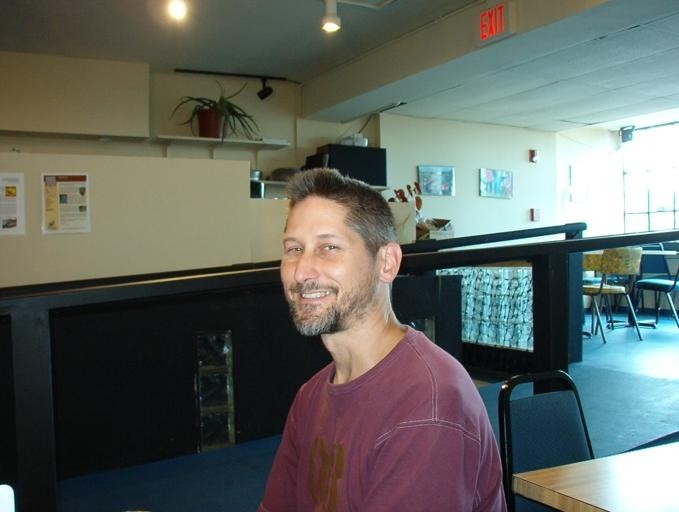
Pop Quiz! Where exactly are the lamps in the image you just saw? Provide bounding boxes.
[320,0,342,33]
[167,0,187,21]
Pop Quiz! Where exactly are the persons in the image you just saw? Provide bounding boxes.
[258,168,508,511]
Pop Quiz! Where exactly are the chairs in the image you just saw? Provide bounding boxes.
[498,370,594,512]
[632,269,679,329]
[582,247,644,344]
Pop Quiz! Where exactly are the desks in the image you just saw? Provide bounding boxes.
[583,250,679,330]
[511,441,678,511]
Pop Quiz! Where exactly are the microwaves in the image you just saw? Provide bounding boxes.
[317,143,387,187]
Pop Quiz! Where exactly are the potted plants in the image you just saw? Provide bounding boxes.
[169,82,260,145]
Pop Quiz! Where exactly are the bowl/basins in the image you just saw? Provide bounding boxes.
[354,137,368,147]
[340,138,354,146]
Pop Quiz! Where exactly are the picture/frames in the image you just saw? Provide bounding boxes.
[417,166,456,196]
[478,167,513,198]
